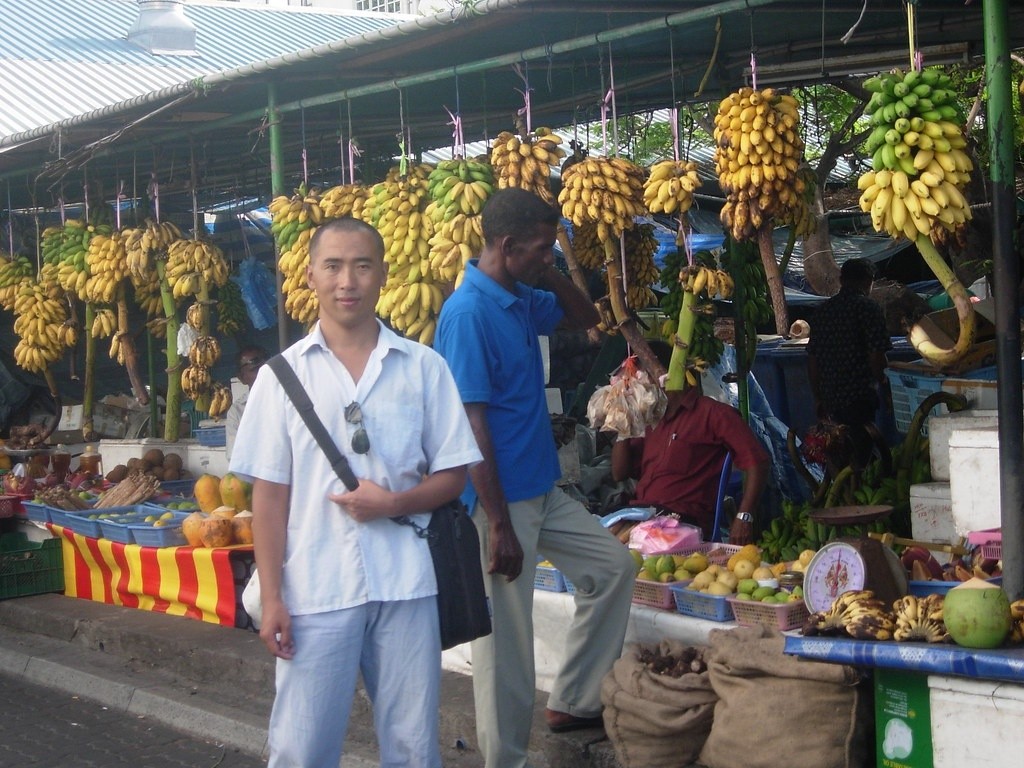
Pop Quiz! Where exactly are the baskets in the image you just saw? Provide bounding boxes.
[529,513,816,630]
[1,467,206,549]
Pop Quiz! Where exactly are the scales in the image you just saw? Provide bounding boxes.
[803,505,908,617]
[3,444,52,479]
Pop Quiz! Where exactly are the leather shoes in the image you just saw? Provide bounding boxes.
[543,706,603,732]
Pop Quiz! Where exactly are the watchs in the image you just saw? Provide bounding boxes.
[736,512,754,523]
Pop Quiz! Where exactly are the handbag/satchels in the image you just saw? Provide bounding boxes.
[425,500,493,649]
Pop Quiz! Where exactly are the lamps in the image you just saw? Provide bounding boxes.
[743,41,979,88]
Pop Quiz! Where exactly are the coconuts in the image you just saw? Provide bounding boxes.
[942,577,1011,649]
[106,449,193,482]
[182,472,254,548]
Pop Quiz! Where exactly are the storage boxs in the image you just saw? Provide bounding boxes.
[536,333,551,385]
[926,674,1024,768]
[57,404,127,439]
[65,504,165,539]
[191,427,226,448]
[545,386,566,415]
[127,517,189,549]
[0,493,18,519]
[909,575,1003,598]
[880,364,997,436]
[533,552,566,593]
[947,424,1001,537]
[871,667,933,768]
[150,498,196,507]
[20,499,50,523]
[635,310,669,343]
[553,427,582,486]
[159,478,194,495]
[928,407,999,481]
[969,526,1003,559]
[667,582,734,623]
[905,307,998,375]
[940,377,998,414]
[96,510,190,544]
[6,492,34,516]
[44,431,84,444]
[561,573,578,594]
[726,591,809,631]
[908,479,958,563]
[633,539,765,610]
[46,500,97,530]
[0,531,66,599]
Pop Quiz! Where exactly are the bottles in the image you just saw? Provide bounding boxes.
[93,474,103,489]
[0,476,5,496]
[79,445,103,484]
[53,443,71,483]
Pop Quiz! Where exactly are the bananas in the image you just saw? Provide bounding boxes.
[799,590,1024,646]
[559,154,776,387]
[266,127,566,348]
[756,435,930,562]
[714,86,819,242]
[0,200,245,416]
[858,69,974,246]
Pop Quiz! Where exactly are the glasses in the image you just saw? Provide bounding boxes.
[239,353,270,371]
[344,399,371,458]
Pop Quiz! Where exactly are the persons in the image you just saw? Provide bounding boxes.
[805,259,893,474]
[225,346,271,462]
[227,218,484,768]
[611,342,770,547]
[434,187,640,768]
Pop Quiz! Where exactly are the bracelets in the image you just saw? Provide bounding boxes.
[554,280,572,295]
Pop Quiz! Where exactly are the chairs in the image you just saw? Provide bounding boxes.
[708,449,735,541]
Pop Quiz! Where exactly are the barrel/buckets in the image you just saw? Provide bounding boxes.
[927,288,977,311]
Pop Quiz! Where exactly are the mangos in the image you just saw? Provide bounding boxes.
[626,545,817,604]
[87,502,199,528]
